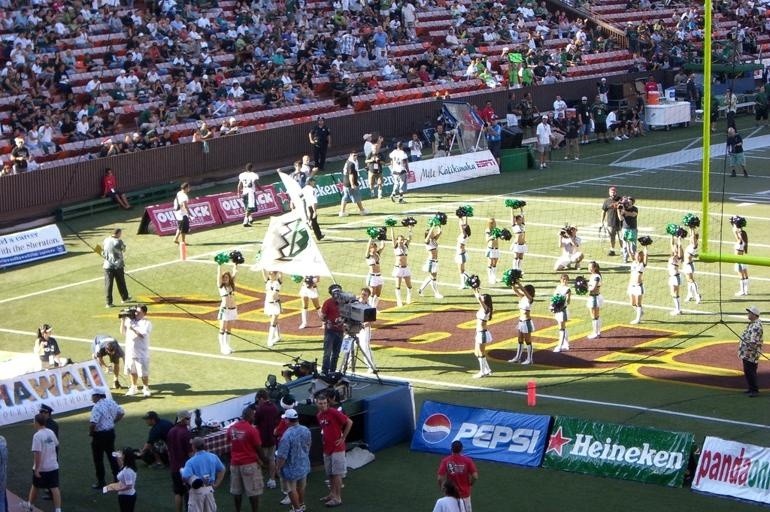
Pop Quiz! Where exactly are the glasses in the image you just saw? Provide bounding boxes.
[353,155,358,156]
[185,416,191,420]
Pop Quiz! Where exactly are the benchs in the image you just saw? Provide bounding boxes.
[718,101,757,121]
[58,180,184,223]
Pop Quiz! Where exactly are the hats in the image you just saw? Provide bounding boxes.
[318,117,325,121]
[542,115,549,119]
[141,411,158,419]
[37,403,54,413]
[40,324,53,333]
[177,410,192,423]
[746,306,761,317]
[363,133,373,141]
[281,409,298,418]
[189,437,205,448]
[89,386,105,394]
[582,96,587,100]
[306,176,316,182]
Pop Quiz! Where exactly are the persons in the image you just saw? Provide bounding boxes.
[237,133,410,241]
[0,1,769,176]
[432,440,478,511]
[217,260,325,355]
[739,307,764,398]
[104,229,132,309]
[601,187,639,261]
[0,389,353,511]
[173,183,194,245]
[367,207,527,314]
[35,324,62,369]
[102,169,134,210]
[473,226,702,379]
[91,303,152,398]
[734,225,750,296]
[321,283,377,378]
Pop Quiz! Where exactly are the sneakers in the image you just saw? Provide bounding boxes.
[142,387,152,396]
[575,158,579,160]
[730,172,736,176]
[539,164,543,171]
[543,163,548,168]
[580,143,585,145]
[339,211,349,217]
[564,157,568,160]
[91,481,107,489]
[121,295,132,303]
[744,173,748,176]
[106,304,115,307]
[399,199,408,204]
[289,505,303,512]
[114,381,121,389]
[391,195,396,203]
[744,389,751,393]
[320,493,335,502]
[279,495,291,504]
[249,219,255,224]
[585,143,590,144]
[371,194,375,198]
[359,208,369,215]
[125,388,138,396]
[318,235,325,240]
[614,136,622,141]
[597,138,601,143]
[378,194,383,199]
[608,250,615,255]
[604,138,611,143]
[621,135,629,140]
[300,504,306,510]
[325,498,342,506]
[18,502,33,512]
[267,479,276,489]
[750,392,760,397]
[243,224,252,227]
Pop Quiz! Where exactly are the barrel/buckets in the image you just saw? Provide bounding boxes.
[665,89,676,102]
[648,91,660,105]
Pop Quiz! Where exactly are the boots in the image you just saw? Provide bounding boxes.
[745,278,749,295]
[268,325,276,347]
[685,282,693,305]
[518,257,525,275]
[396,288,403,307]
[521,343,534,365]
[552,328,566,353]
[512,258,518,269]
[457,274,464,288]
[273,323,281,344]
[492,266,496,284]
[224,332,235,353]
[475,354,492,375]
[373,295,380,314]
[472,355,486,379]
[316,306,326,330]
[369,295,374,308]
[691,282,701,304]
[298,307,309,329]
[562,329,569,351]
[219,332,231,354]
[587,318,598,339]
[507,342,524,363]
[487,266,492,283]
[630,307,642,324]
[418,276,431,295]
[431,278,443,298]
[632,304,644,317]
[735,279,745,296]
[406,287,412,304]
[597,316,601,336]
[670,296,681,316]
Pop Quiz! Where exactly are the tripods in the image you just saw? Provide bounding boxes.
[339,340,387,385]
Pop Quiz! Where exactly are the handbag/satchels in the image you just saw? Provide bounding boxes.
[174,197,180,211]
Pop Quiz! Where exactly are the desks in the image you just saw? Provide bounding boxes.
[644,100,692,132]
[184,424,232,466]
